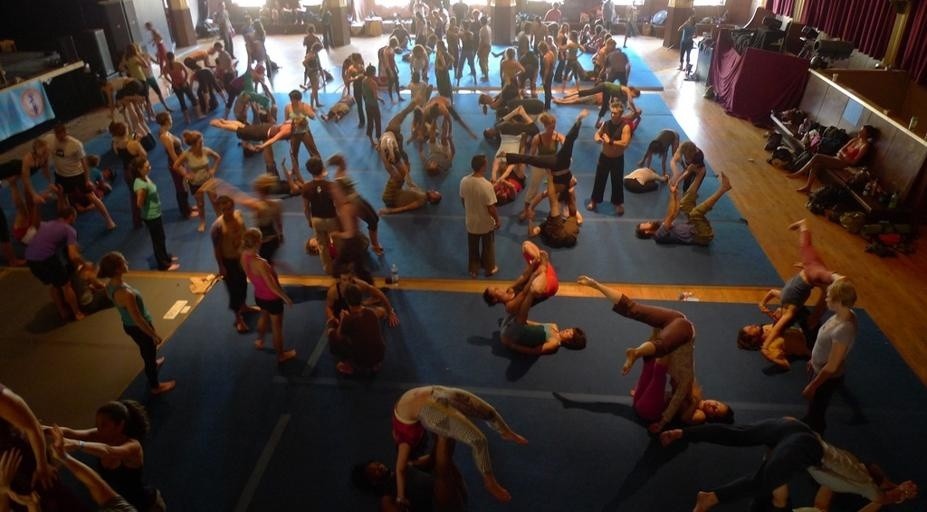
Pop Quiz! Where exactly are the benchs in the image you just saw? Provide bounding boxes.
[350,16,413,35]
[516,19,744,36]
[231,20,320,34]
[769,108,898,215]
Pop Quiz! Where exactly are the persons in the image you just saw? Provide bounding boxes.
[797,282,864,431]
[349,385,530,512]
[2,1,925,373]
[661,416,923,512]
[1,384,166,512]
[577,276,735,445]
[97,250,176,395]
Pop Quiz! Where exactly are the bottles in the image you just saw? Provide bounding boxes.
[848,167,901,210]
